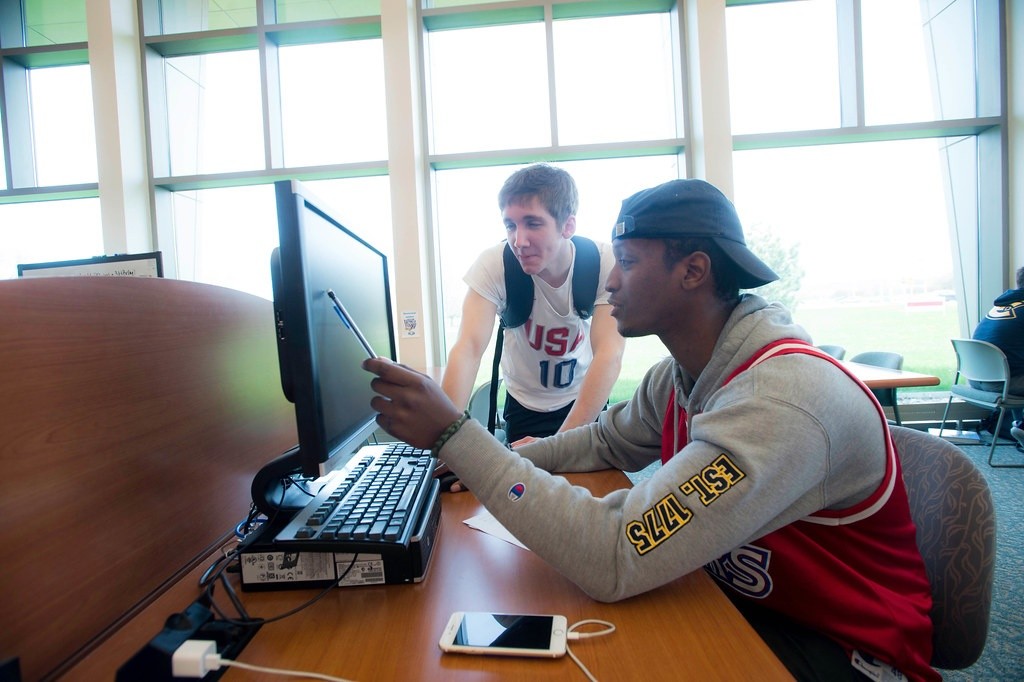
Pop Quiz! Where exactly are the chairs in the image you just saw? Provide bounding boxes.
[890,424,997,670]
[816,345,846,362]
[848,351,904,426]
[939,337,1024,468]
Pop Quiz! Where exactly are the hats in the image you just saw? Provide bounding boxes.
[612,179,780,290]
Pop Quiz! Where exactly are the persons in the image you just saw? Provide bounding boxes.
[969,266,1024,451]
[438,166,629,493]
[358,180,948,682]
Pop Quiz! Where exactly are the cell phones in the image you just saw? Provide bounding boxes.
[438,612,568,658]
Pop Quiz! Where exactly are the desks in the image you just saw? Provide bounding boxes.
[51,470,794,682]
[834,360,941,388]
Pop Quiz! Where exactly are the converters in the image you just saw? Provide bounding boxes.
[150,602,214,660]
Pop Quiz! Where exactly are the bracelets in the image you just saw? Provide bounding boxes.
[430,409,472,457]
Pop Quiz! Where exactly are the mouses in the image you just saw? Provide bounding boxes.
[439,471,460,493]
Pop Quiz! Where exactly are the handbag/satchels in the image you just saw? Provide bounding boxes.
[986,409,1024,442]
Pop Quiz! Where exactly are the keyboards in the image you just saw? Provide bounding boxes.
[273,444,437,551]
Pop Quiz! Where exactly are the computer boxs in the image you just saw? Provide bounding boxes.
[238,479,442,592]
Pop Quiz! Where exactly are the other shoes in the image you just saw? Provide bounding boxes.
[1011,420,1024,449]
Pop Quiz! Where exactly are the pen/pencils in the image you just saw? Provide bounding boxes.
[327,289,377,359]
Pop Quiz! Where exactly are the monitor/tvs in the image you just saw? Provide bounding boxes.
[250,178,401,520]
[18,252,164,279]
